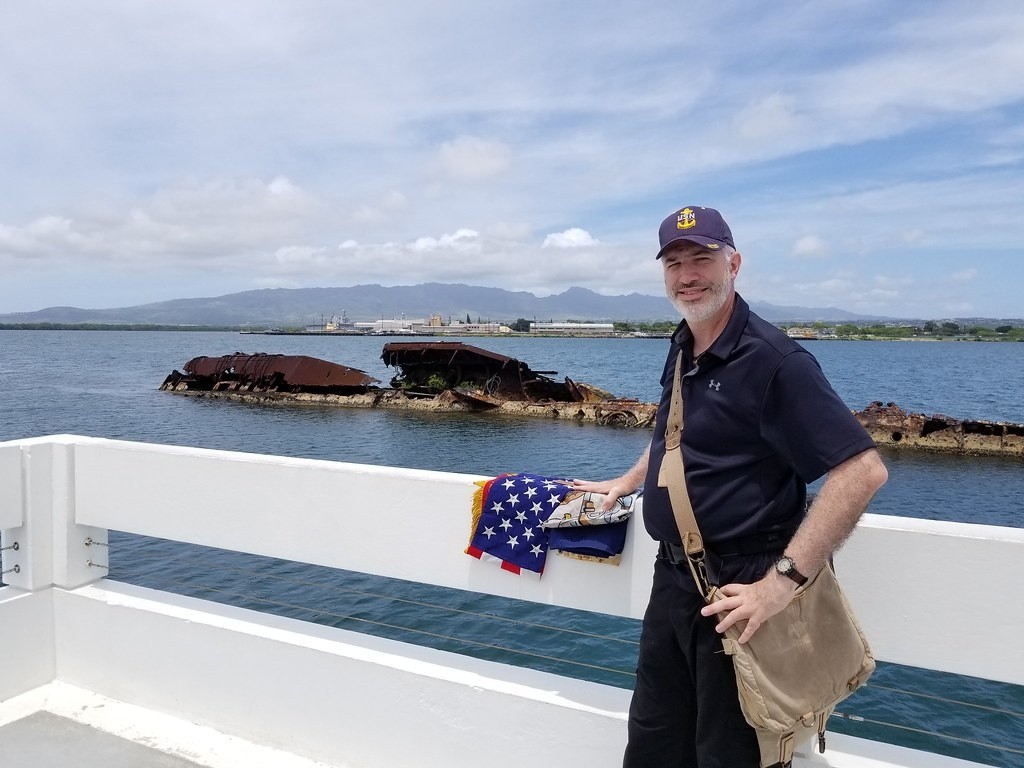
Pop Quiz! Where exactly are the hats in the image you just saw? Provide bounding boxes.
[655,206,736,260]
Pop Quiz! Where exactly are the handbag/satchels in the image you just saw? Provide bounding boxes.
[707,562,876,768]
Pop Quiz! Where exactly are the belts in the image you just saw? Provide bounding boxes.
[657,537,786,565]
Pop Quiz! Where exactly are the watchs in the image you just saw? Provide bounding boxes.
[775,555,809,585]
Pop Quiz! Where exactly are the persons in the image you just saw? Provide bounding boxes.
[571,206,889,768]
